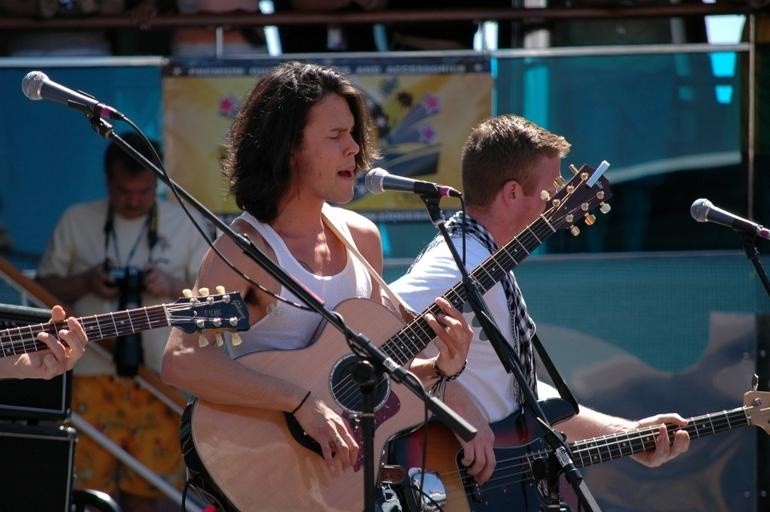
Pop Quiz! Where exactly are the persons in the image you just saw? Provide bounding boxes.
[33,132,216,511]
[381,110,692,510]
[161,62,475,512]
[0,304,87,380]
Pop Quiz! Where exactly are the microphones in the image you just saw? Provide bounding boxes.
[690,197,770,240]
[21,71,124,122]
[364,166,462,199]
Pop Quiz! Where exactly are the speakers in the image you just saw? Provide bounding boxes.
[0,423,77,512]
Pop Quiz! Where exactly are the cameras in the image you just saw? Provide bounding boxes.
[102,267,151,380]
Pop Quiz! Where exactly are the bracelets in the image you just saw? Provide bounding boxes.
[290,390,313,416]
[433,357,467,385]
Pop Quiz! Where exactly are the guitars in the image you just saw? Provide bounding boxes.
[179,160,613,512]
[374,373,770,511]
[0,284,249,358]
